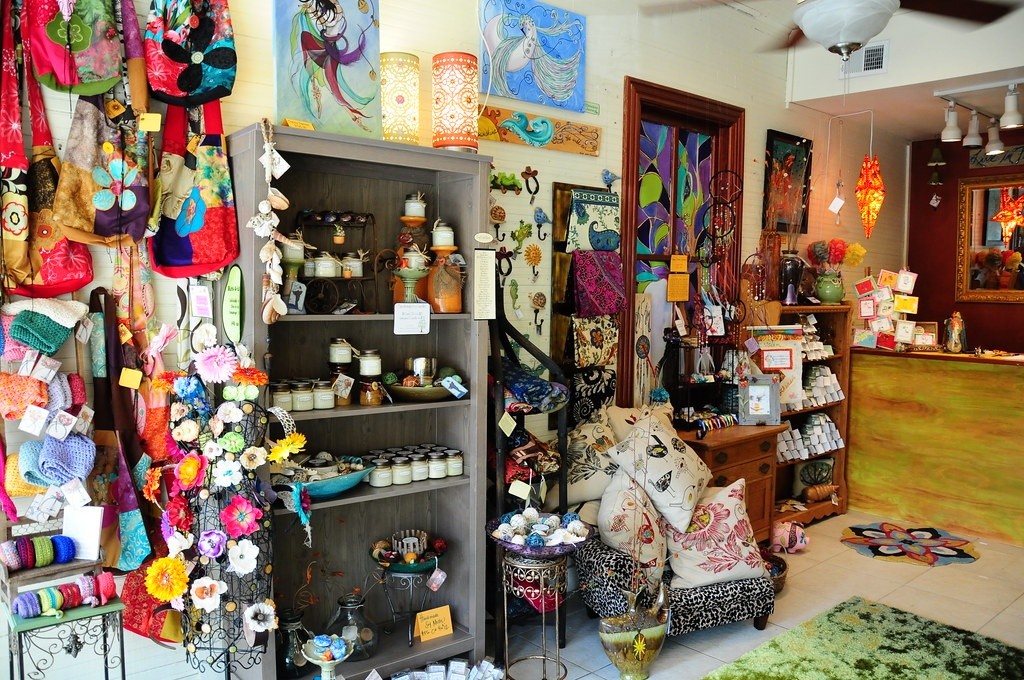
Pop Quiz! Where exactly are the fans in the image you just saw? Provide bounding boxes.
[641,0,1020,51]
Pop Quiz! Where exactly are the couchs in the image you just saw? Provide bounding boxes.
[577,538,775,642]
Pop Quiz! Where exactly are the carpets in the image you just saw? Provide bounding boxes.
[704,595,1024,680]
[841,521,979,566]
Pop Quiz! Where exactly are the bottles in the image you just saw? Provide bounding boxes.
[265,230,465,502]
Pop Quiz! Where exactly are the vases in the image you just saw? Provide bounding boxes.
[598,580,671,680]
[817,270,844,308]
[978,270,1019,290]
[780,250,802,305]
[759,229,781,299]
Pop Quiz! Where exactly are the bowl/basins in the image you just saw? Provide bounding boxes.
[284,463,377,500]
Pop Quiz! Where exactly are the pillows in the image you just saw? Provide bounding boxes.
[534,407,766,588]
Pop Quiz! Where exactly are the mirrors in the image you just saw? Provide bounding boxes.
[953,174,1024,303]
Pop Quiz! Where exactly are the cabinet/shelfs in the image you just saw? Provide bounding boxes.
[292,208,382,317]
[674,264,851,543]
[229,121,491,680]
[1,560,126,680]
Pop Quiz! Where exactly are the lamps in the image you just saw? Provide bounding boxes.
[791,0,901,63]
[940,84,1023,155]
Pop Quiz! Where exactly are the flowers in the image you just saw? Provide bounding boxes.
[147,344,308,632]
[967,248,1023,272]
[807,239,866,275]
[584,385,690,595]
[763,130,821,252]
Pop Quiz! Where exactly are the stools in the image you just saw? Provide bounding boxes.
[501,551,567,680]
[382,570,433,647]
